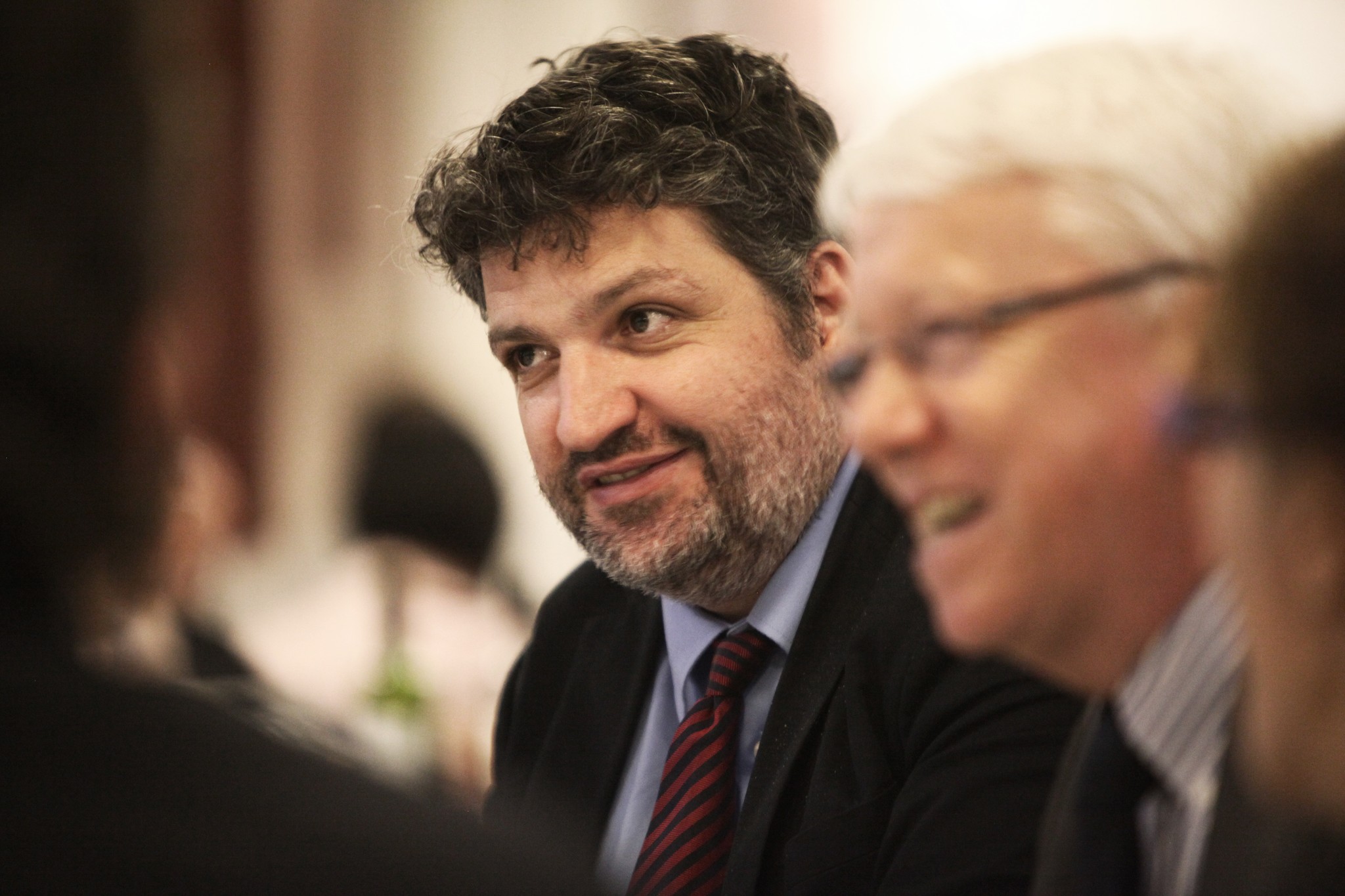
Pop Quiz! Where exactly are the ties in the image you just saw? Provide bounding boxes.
[1069,707,1162,896]
[629,627,775,896]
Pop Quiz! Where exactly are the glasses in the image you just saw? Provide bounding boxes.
[826,260,1228,434]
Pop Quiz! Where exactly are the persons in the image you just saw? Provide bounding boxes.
[408,31,1070,895]
[0,1,1345,896]
